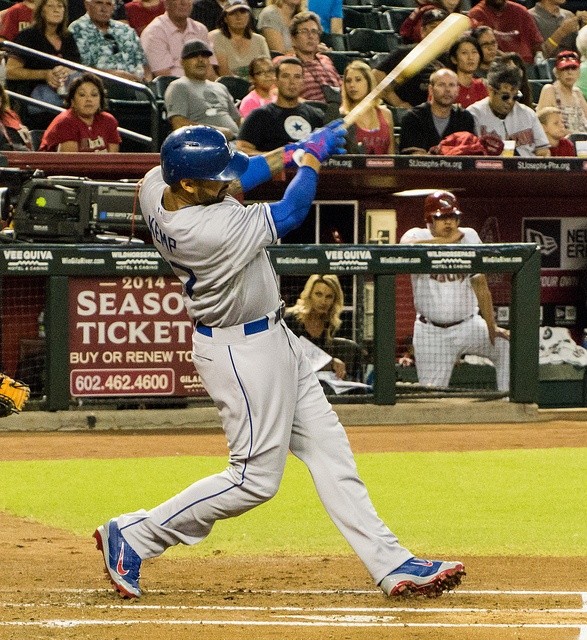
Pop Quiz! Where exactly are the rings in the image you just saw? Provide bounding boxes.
[342,371,345,373]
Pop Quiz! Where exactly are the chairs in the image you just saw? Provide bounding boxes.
[108,98,151,134]
[565,133,587,157]
[326,335,364,382]
[318,0,559,110]
[146,74,181,140]
[216,75,252,101]
[29,128,47,150]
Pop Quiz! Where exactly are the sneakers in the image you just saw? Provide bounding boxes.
[93,519,142,599]
[381,558,467,600]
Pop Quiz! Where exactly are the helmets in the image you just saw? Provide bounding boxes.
[161,125,250,184]
[425,191,463,218]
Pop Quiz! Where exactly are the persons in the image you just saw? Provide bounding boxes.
[526,0,586,60]
[4,0,82,115]
[91,117,467,602]
[139,0,221,82]
[0,83,35,151]
[535,106,576,156]
[487,52,535,111]
[370,8,456,112]
[535,50,586,135]
[281,274,346,380]
[399,0,463,42]
[37,71,122,151]
[0,0,42,41]
[235,57,324,158]
[270,11,342,104]
[255,0,306,56]
[337,59,394,154]
[111,0,127,21]
[399,68,477,155]
[426,36,489,109]
[469,25,498,79]
[67,0,152,102]
[163,37,241,142]
[467,0,543,64]
[189,0,227,32]
[207,0,272,82]
[238,55,278,122]
[307,0,348,52]
[124,0,166,37]
[398,190,510,393]
[465,61,550,156]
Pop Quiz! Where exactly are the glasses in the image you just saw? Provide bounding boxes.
[487,85,523,101]
[253,69,275,76]
[294,28,321,36]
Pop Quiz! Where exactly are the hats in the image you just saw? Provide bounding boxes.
[422,9,448,25]
[224,1,251,13]
[182,39,213,59]
[555,51,580,70]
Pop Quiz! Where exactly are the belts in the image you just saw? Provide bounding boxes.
[197,301,286,338]
[419,315,474,328]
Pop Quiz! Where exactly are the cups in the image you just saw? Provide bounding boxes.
[502,139,516,157]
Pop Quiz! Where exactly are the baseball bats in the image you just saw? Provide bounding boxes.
[293,12,472,170]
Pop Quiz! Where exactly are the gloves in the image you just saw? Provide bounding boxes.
[284,119,348,169]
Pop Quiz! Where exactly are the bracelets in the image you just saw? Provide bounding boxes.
[547,37,557,46]
